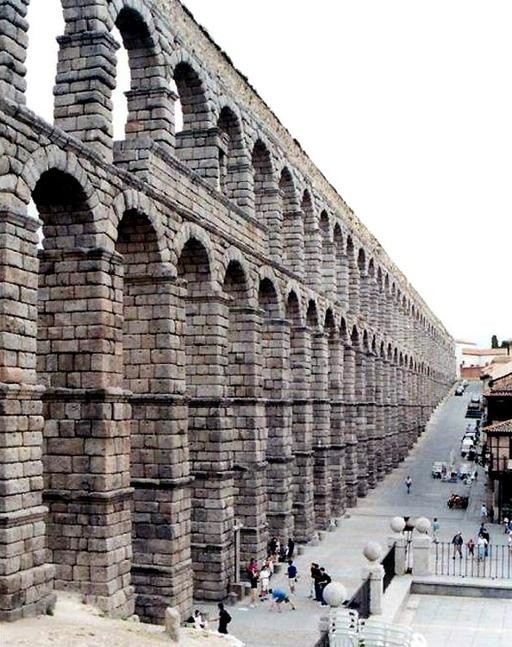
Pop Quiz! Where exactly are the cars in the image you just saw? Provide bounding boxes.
[454,378,470,396]
[431,461,478,481]
[468,392,480,411]
[460,423,480,457]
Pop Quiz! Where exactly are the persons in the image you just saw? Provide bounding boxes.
[254,536,287,597]
[477,521,489,560]
[261,566,270,601]
[451,531,463,558]
[502,515,512,542]
[216,602,231,632]
[268,588,295,612]
[287,559,296,595]
[288,537,294,557]
[404,475,412,493]
[191,609,203,628]
[248,557,257,577]
[315,566,331,605]
[311,562,319,600]
[432,517,441,543]
[467,538,475,555]
[479,504,488,519]
